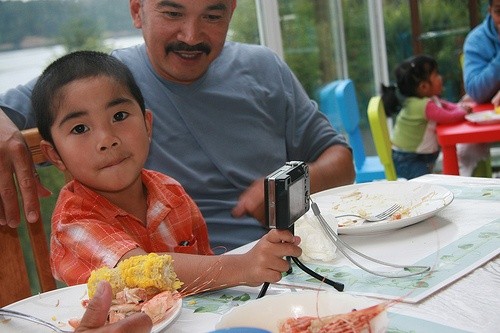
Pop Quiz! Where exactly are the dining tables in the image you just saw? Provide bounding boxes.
[435,103,500,178]
[0,171,500,333]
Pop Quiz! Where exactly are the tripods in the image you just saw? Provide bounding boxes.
[256,223,344,299]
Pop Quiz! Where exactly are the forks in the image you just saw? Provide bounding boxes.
[335,204,401,222]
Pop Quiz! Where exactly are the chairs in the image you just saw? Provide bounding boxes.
[367,97,396,180]
[318,78,385,184]
[0,127,56,307]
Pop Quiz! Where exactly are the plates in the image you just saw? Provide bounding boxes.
[464,109,500,126]
[0,283,183,333]
[309,180,454,235]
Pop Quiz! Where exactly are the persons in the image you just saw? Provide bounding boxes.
[0,0,356,254]
[381,56,473,179]
[457,0,500,177]
[31,50,301,292]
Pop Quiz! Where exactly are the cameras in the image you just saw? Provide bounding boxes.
[264,161,310,229]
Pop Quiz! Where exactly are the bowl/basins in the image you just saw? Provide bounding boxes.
[215,289,391,333]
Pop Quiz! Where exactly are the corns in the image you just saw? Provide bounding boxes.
[87,252,184,300]
